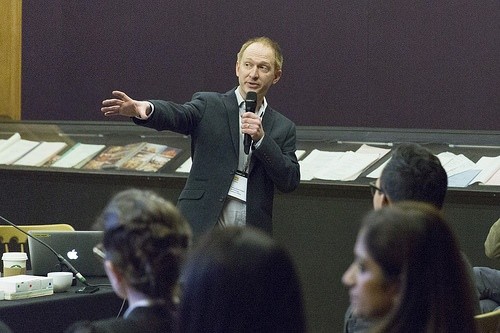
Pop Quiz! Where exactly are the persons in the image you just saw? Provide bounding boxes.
[175,224,307,333]
[342,144,500,333]
[101,35,301,246]
[87,189,192,333]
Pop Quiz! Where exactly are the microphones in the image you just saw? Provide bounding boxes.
[244,91,257,155]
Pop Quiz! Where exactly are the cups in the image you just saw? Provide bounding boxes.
[47,272,73,292]
[2,252,28,276]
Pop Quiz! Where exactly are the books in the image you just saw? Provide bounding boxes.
[0,133,180,174]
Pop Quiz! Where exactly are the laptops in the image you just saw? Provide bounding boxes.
[27,229,109,277]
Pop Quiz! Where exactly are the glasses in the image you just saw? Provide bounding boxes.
[93,243,107,260]
[369,181,384,196]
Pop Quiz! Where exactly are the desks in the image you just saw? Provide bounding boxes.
[0,276,129,333]
